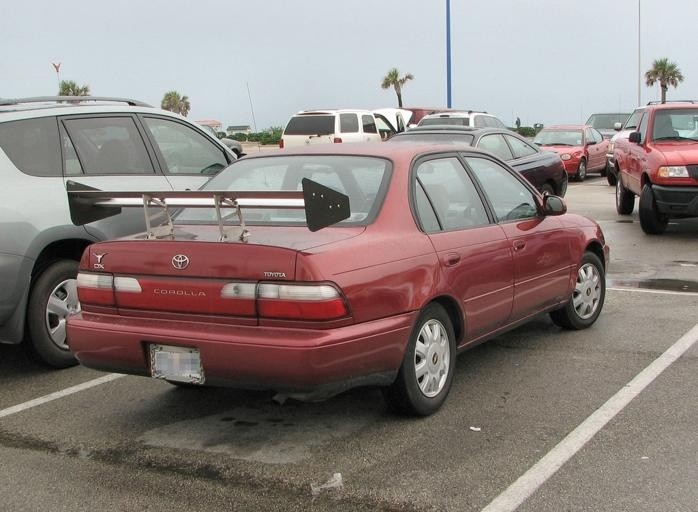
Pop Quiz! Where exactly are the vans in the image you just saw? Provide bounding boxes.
[578,112,632,145]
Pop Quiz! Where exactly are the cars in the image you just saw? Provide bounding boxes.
[154,123,243,173]
[386,127,568,212]
[64,139,610,420]
[532,124,609,181]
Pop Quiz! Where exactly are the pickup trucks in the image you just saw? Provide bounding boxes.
[612,100,698,235]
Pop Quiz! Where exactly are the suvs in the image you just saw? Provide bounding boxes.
[278,108,413,150]
[605,102,698,185]
[1,95,242,368]
[409,109,506,129]
